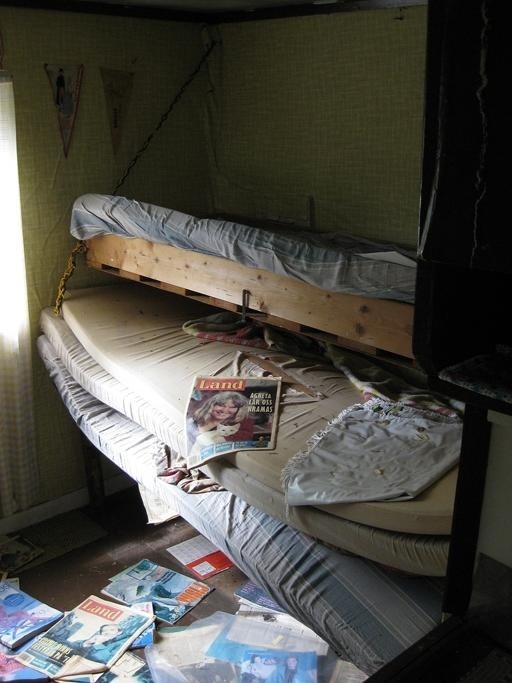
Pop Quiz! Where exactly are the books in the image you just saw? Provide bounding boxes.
[0,571,156,683]
[184,372,284,471]
[102,558,214,625]
[233,576,290,615]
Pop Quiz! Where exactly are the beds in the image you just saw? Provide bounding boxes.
[38,197,508,631]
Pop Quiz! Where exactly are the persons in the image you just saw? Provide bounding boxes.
[189,389,257,450]
[241,655,279,683]
[267,656,313,683]
